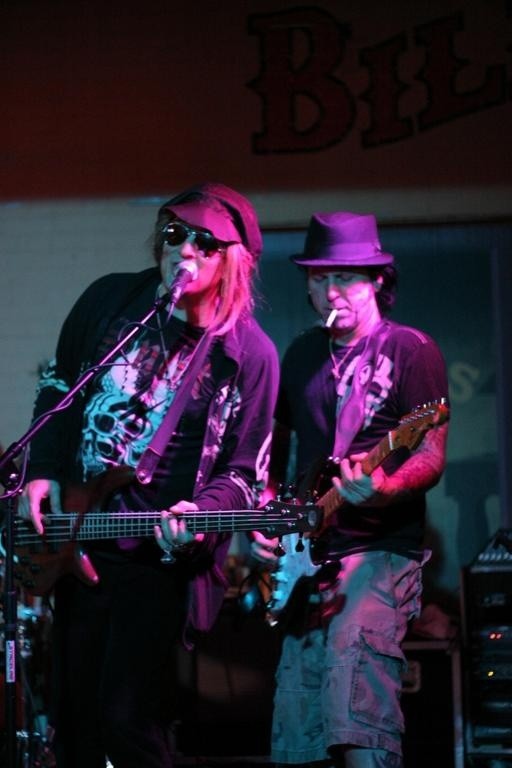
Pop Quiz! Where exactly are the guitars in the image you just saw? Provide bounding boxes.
[0,452,324,597]
[247,397,450,639]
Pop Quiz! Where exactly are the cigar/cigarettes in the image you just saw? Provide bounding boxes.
[325,309,337,327]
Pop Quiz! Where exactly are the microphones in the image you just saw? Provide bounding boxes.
[168,261,198,304]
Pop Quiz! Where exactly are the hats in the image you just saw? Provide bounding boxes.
[289,212,394,266]
[159,181,263,257]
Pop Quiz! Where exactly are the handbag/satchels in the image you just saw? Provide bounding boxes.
[412,604,450,639]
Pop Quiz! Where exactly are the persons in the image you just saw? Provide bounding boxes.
[250,211,450,768]
[18,183,281,768]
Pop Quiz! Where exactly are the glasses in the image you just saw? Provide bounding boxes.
[158,222,239,250]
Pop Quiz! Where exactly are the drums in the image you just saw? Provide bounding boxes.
[0,602,49,675]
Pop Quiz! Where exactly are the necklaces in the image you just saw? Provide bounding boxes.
[155,292,207,390]
[329,336,358,379]
